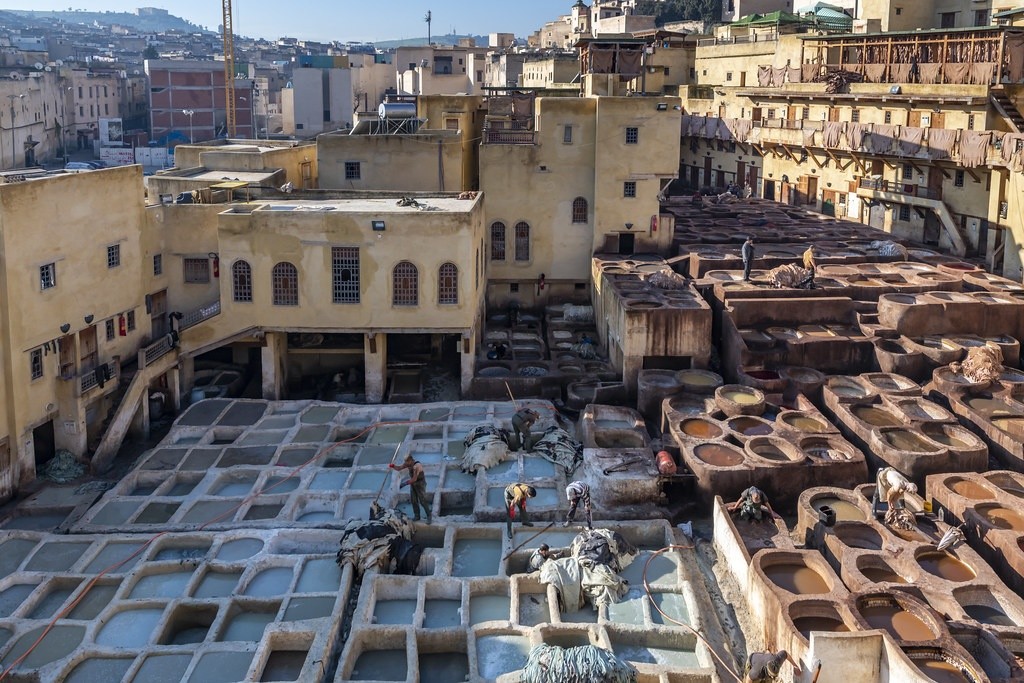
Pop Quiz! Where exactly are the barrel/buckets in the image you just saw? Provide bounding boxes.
[923,502,933,513]
[191,387,206,403]
[149,397,163,420]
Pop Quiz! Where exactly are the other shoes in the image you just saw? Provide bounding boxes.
[412,515,420,521]
[523,521,533,526]
[508,530,512,538]
[526,448,536,453]
[588,521,593,529]
[563,520,572,527]
[872,513,877,519]
[426,514,432,524]
[517,443,523,448]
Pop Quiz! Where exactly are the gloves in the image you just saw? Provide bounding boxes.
[389,464,394,467]
[400,482,408,488]
[522,502,526,509]
[510,509,515,518]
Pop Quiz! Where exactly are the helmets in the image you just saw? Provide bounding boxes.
[540,544,550,551]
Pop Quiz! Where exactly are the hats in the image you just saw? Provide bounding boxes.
[536,411,540,419]
[752,493,760,503]
[566,487,577,500]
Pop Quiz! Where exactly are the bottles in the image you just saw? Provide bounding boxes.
[938,507,944,521]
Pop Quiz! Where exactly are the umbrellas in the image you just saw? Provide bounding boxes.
[937,519,969,552]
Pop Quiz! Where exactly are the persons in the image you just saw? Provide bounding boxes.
[871,467,918,519]
[512,408,540,453]
[581,332,587,341]
[527,543,553,573]
[563,481,593,529]
[742,236,753,281]
[727,181,734,194]
[801,245,817,289]
[744,650,801,683]
[389,454,432,524]
[729,486,782,519]
[504,483,536,539]
